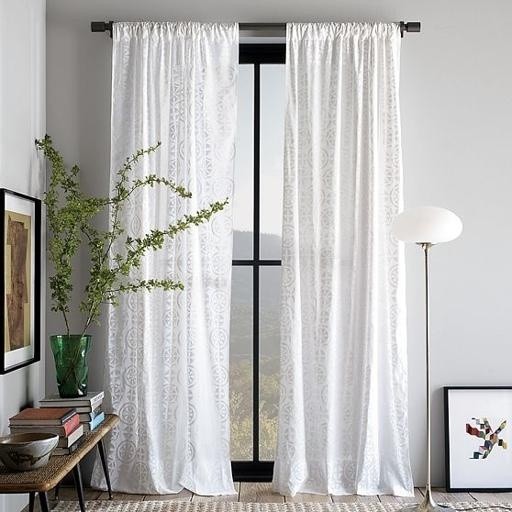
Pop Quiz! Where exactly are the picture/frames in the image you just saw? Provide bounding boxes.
[1,188,42,374]
[443,385,512,492]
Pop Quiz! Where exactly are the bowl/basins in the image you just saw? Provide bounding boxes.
[0,432,61,472]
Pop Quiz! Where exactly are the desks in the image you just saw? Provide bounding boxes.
[0,413,120,512]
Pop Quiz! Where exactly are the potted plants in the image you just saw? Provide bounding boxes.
[35,132,230,398]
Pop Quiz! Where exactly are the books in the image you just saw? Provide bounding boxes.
[8,390,106,458]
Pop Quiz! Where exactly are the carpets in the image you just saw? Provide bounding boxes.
[47,498,511,511]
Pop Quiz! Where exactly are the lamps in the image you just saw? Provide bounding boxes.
[390,202,461,511]
